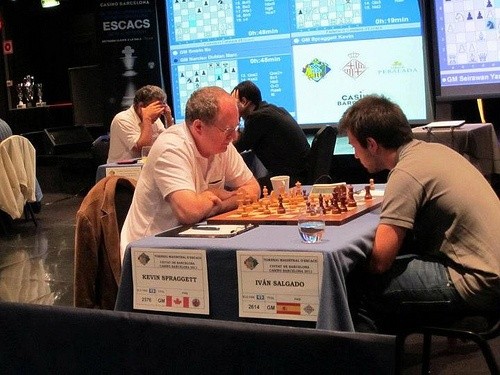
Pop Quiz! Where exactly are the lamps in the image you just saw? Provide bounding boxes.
[41,0,60,8]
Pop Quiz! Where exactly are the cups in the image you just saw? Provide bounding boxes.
[270,176,290,194]
[296,206,325,243]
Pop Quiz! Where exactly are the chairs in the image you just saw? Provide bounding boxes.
[303,125,338,184]
[394,302,500,375]
[75,176,138,309]
[0,135,43,240]
[92,135,111,166]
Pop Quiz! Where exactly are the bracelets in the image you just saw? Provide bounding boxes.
[166,118,173,124]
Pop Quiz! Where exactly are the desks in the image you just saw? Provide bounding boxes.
[96,158,144,185]
[114,184,386,336]
[412,123,500,176]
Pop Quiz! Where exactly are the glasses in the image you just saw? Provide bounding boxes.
[194,115,239,136]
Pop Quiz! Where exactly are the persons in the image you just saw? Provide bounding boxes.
[231,81,312,197]
[107,84,173,164]
[0,120,12,143]
[120,85,260,272]
[337,93,500,333]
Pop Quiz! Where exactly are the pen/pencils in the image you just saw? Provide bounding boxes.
[192,227,220,230]
